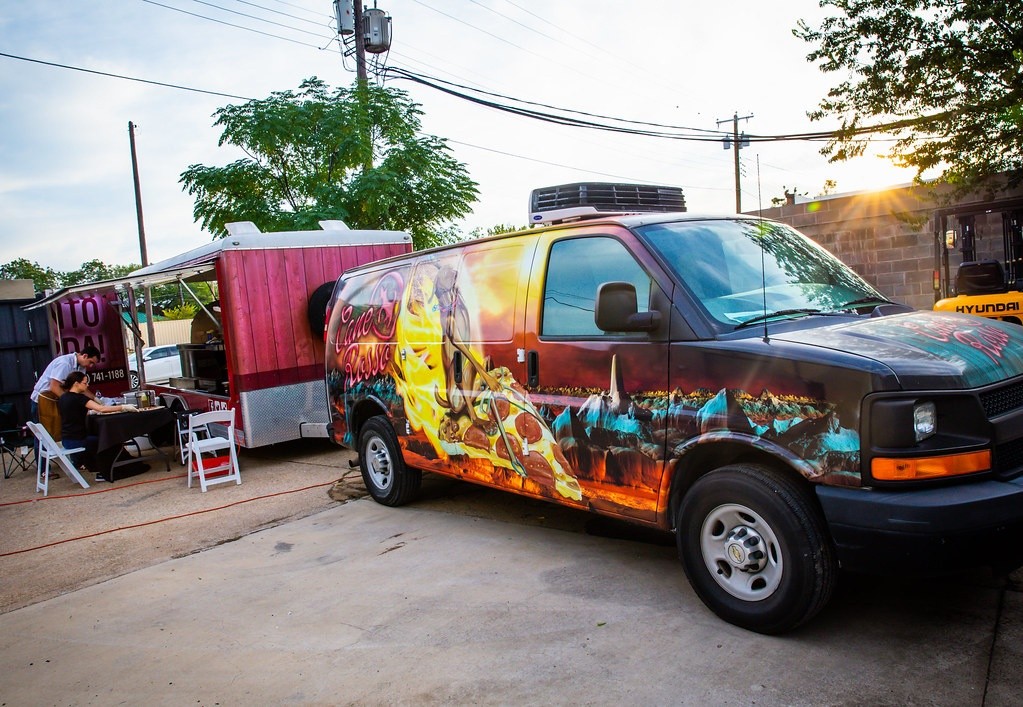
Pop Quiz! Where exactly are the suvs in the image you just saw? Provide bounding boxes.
[323,182,1023,639]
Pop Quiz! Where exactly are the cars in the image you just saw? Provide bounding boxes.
[128,344,182,390]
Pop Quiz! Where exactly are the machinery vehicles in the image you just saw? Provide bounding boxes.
[929,196,1023,326]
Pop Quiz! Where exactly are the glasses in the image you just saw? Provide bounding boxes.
[80,381,88,386]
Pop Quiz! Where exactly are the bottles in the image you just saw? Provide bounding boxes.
[95,389,101,399]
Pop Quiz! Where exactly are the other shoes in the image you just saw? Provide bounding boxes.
[74,464,86,470]
[40,471,58,479]
[94,473,105,482]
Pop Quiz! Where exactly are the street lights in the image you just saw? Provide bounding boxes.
[715,111,755,214]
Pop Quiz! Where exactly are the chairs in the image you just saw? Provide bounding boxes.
[174,411,218,465]
[186,407,241,493]
[26,421,91,497]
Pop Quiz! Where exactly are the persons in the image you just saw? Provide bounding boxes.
[56,372,138,482]
[30,346,102,480]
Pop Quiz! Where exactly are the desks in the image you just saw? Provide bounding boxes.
[87,405,172,483]
